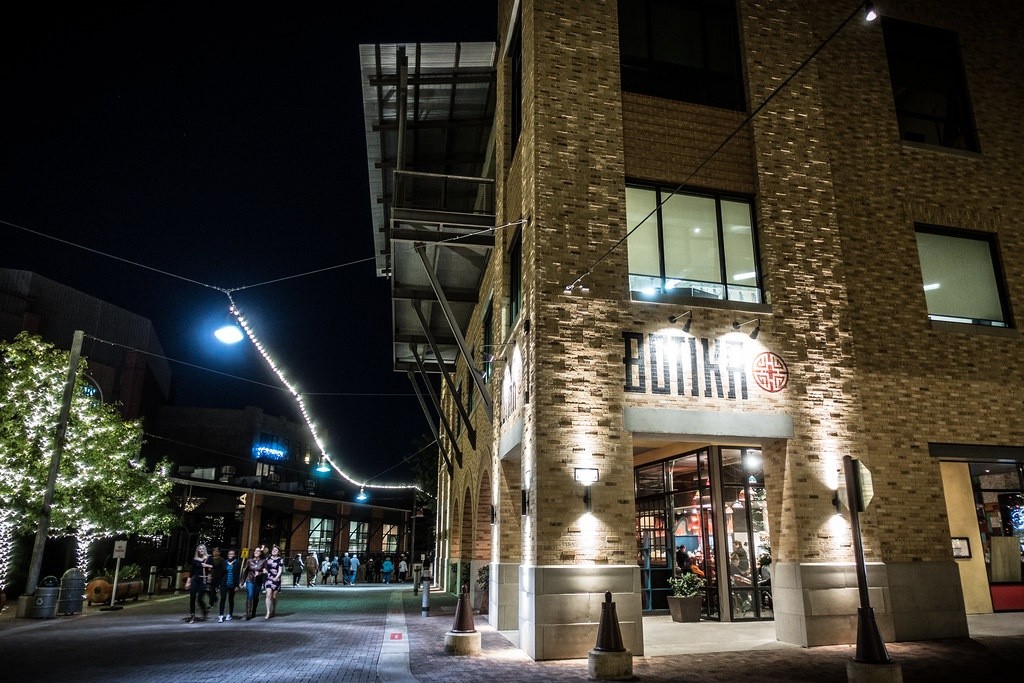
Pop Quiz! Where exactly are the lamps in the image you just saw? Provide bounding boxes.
[215,312,244,345]
[357,488,366,499]
[585,274,600,296]
[521,490,529,516]
[489,505,496,525]
[669,311,692,333]
[438,529,450,540]
[316,456,331,471]
[477,339,516,362]
[832,490,843,516]
[472,357,507,378]
[582,485,593,513]
[866,2,877,21]
[733,318,761,339]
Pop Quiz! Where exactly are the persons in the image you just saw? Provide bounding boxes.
[374,555,384,583]
[340,552,352,585]
[349,553,360,585]
[731,540,749,571]
[330,556,339,585]
[292,554,305,588]
[321,557,330,584]
[729,553,752,605]
[238,545,269,621]
[304,551,319,588]
[364,559,375,583]
[185,543,216,624]
[426,550,429,556]
[207,547,226,608]
[398,559,408,583]
[265,544,284,620]
[259,544,268,558]
[757,552,772,610]
[676,544,705,579]
[380,557,395,584]
[215,549,241,623]
[430,550,434,563]
[423,556,430,570]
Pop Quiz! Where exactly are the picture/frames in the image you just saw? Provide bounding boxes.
[951,537,971,558]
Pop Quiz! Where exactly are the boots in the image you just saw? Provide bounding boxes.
[246,600,251,620]
[252,600,258,618]
[189,615,195,623]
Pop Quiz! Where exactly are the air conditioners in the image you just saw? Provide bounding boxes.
[305,480,316,487]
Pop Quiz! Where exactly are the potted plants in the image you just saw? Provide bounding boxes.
[85,563,144,607]
[450,562,471,594]
[665,572,705,622]
[476,565,489,614]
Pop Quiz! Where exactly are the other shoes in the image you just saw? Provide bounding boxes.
[293,586,297,588]
[309,582,314,587]
[296,584,299,587]
[265,612,270,619]
[218,616,223,622]
[225,615,232,620]
[270,611,275,618]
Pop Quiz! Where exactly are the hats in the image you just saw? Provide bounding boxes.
[370,559,373,562]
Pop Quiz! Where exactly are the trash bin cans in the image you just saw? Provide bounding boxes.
[32,576,59,618]
[56,568,88,616]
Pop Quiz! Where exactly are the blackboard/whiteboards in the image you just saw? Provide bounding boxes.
[160,577,170,590]
